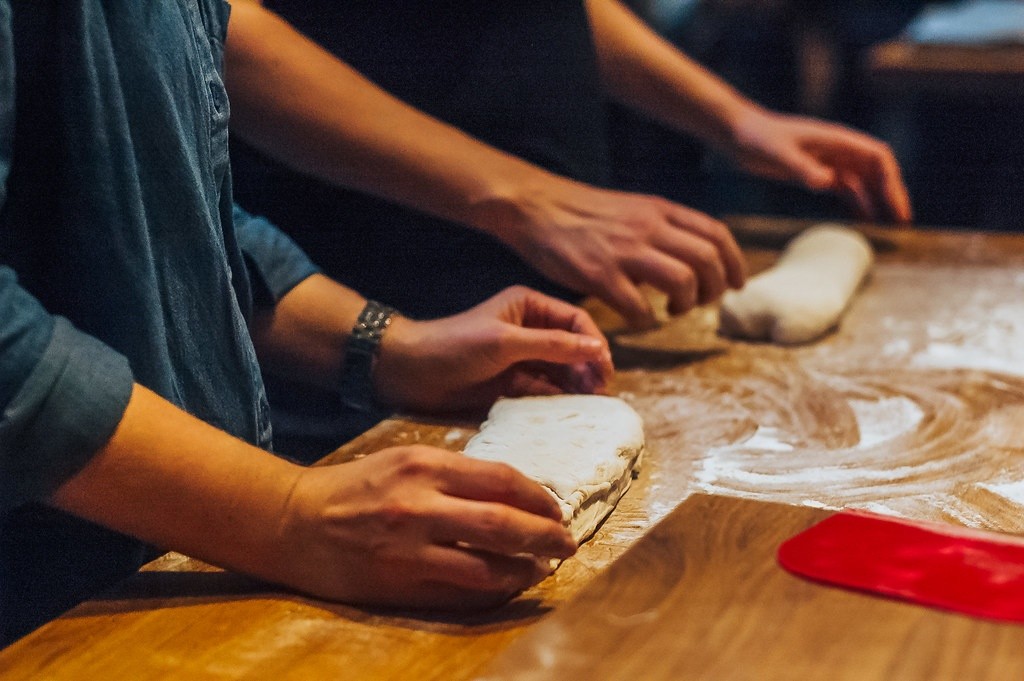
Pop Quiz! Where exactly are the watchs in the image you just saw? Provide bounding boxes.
[340,298,399,412]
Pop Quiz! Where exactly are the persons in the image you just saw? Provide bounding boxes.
[227,0,912,466]
[0,0,615,650]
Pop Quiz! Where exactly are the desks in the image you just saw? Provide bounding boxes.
[1,249,1024,681]
